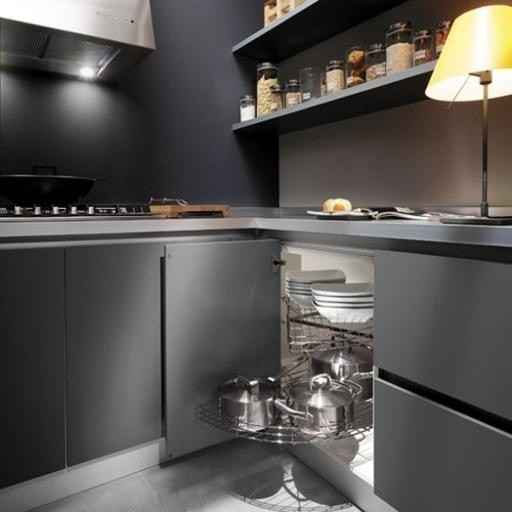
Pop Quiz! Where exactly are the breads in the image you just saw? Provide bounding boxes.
[321,197,353,213]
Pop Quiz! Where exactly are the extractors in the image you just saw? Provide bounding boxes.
[0,0,156,83]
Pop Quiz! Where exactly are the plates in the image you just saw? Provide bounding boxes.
[306,209,379,218]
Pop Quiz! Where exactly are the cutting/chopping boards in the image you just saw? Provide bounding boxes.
[148,204,230,218]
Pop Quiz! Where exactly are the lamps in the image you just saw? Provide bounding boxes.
[424,4,512,225]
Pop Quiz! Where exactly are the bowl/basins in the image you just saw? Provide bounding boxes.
[284,267,374,324]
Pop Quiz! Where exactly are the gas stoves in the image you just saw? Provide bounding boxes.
[0,203,151,218]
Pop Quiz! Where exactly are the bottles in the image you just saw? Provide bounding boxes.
[239,20,451,122]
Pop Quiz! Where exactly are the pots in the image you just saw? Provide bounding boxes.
[212,333,374,436]
[0,164,108,207]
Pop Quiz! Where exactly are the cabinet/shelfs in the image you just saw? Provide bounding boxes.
[163,232,371,508]
[371,246,512,509]
[230,0,437,138]
[2,241,164,510]
